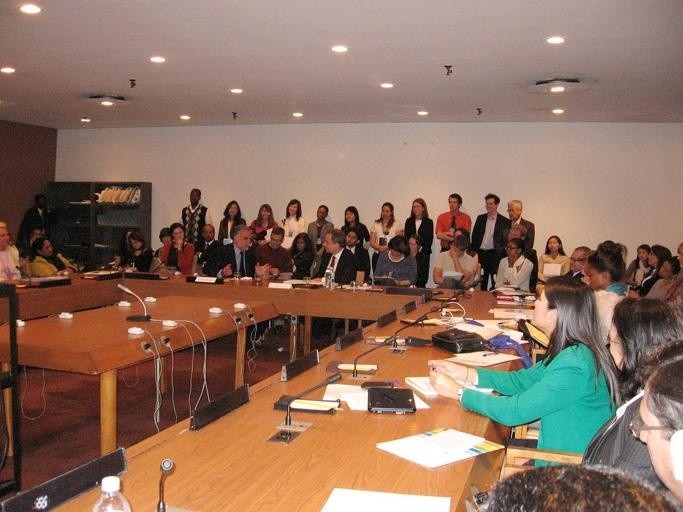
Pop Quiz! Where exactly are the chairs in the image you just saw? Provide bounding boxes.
[500,348,586,483]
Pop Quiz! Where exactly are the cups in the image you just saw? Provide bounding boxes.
[234,272,241,281]
[303,277,311,284]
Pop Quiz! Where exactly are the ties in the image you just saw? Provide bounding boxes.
[237,250,247,278]
[330,255,336,268]
[449,215,458,228]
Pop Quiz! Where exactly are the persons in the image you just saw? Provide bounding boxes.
[0,222,19,283]
[632,351,682,503]
[30,237,74,277]
[23,229,41,267]
[427,275,623,468]
[578,294,682,479]
[481,459,674,511]
[16,195,50,250]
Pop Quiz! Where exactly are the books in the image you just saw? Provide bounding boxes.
[96,185,139,206]
[404,371,494,397]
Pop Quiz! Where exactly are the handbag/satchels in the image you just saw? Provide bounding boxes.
[432,328,491,356]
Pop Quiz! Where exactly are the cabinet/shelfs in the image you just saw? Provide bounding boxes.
[44,180,92,267]
[92,181,152,261]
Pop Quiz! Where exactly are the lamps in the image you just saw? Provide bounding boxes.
[86,94,127,107]
[524,78,588,95]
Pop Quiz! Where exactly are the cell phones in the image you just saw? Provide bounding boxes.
[361,382,392,389]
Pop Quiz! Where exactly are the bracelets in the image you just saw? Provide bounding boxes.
[457,384,465,402]
[464,363,470,383]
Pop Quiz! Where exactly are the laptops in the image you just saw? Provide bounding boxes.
[368,388,416,414]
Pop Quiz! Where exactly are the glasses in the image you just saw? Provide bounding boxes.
[504,245,518,251]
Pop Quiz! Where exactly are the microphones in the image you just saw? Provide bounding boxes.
[389,272,485,353]
[157,458,175,512]
[275,373,341,432]
[16,266,36,288]
[117,283,151,321]
[346,336,395,381]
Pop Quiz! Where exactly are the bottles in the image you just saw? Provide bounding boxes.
[325,266,335,290]
[92,475,131,512]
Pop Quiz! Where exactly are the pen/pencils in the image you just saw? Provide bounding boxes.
[375,391,391,401]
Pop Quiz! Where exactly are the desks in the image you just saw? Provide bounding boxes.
[1,268,536,512]
